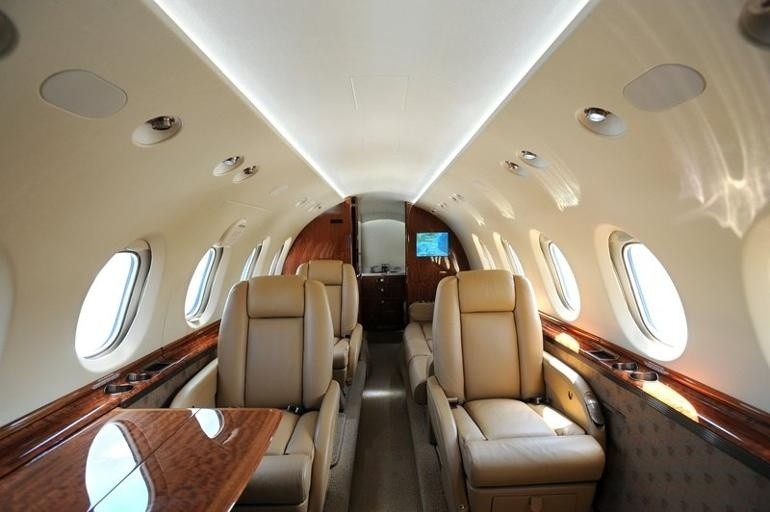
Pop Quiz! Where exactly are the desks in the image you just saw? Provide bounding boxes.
[0,401,283,512]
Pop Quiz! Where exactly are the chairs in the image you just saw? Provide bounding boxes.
[424,271,610,510]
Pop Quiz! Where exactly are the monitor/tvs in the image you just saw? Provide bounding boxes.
[415,231,449,257]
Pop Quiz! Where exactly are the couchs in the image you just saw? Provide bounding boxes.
[403,302,435,405]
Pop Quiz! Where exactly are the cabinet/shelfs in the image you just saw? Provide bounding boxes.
[362,276,405,332]
